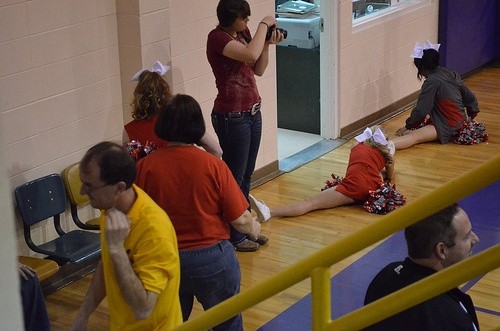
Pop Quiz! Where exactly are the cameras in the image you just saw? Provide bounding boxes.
[265,23,288,41]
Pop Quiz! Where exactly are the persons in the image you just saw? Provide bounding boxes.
[18,262,50,331]
[67,141,184,331]
[365,204,480,331]
[122,62,223,163]
[391,40,480,149]
[249,126,394,222]
[206,0,284,252]
[134,95,261,331]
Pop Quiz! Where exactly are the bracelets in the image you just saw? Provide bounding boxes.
[260,22,268,26]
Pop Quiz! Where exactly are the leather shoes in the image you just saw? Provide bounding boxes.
[254,236,268,245]
[234,238,260,252]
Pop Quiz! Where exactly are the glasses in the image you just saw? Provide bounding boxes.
[84,184,107,194]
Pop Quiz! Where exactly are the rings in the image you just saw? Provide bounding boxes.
[21,265,25,269]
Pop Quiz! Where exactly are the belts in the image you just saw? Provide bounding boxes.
[243,102,261,115]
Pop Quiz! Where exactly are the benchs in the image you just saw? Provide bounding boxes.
[15,161,102,296]
